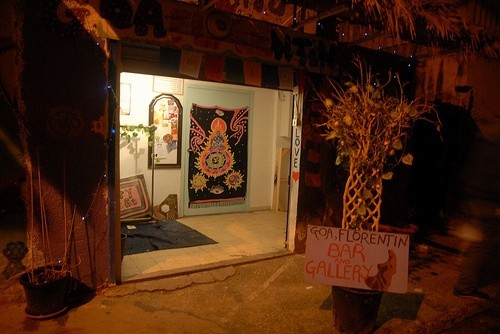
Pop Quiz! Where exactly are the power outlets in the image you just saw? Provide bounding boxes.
[292,114,301,127]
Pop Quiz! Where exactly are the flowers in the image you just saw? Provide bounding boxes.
[311,57,443,231]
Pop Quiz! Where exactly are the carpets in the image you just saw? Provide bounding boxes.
[121,218,219,256]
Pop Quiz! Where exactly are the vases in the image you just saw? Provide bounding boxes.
[19,264,73,316]
[364,221,419,258]
[330,285,384,334]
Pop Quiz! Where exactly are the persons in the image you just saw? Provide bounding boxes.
[163,133,177,153]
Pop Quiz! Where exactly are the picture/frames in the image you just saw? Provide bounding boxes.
[148,93,183,169]
